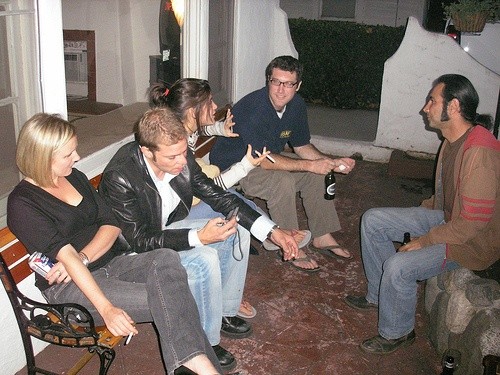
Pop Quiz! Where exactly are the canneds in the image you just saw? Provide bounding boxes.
[28,251,55,278]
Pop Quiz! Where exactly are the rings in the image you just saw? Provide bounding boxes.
[55,270,61,276]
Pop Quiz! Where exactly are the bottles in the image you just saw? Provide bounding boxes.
[401,232,411,246]
[324,169,336,200]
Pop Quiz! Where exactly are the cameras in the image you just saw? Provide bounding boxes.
[221,205,241,226]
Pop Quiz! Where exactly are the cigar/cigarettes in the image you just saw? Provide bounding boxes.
[263,152,276,163]
[292,254,299,259]
[125,327,136,344]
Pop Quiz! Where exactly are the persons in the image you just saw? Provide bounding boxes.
[208,56,356,272]
[5,113,220,375]
[96,111,298,371]
[344,74,500,354]
[144,76,311,319]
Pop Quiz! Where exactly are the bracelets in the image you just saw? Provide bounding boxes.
[78,251,90,267]
[266,225,279,237]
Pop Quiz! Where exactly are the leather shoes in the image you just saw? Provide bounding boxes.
[220,315,253,339]
[345,294,379,311]
[359,329,415,354]
[210,345,237,371]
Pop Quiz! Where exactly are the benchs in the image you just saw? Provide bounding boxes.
[0,103,233,375]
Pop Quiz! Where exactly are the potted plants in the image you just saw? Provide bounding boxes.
[442,0,500,36]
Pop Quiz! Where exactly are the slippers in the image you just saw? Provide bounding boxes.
[236,300,257,318]
[308,242,351,260]
[263,229,311,251]
[277,250,320,272]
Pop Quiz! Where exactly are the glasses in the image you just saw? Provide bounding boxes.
[268,75,298,88]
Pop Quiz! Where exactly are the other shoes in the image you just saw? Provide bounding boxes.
[174,364,198,375]
